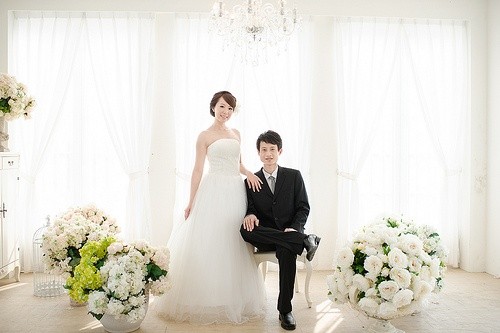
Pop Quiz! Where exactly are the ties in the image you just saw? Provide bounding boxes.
[268,177,276,195]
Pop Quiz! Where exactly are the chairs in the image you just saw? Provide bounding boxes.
[246,230,313,308]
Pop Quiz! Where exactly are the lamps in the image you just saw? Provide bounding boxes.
[206,0,298,70]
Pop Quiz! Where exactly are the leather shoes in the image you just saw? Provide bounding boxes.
[279,313,296,330]
[304,234,321,261]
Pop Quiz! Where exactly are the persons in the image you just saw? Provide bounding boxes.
[156,91,267,325]
[240,130,322,331]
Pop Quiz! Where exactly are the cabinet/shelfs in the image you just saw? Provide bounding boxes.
[0,151,21,282]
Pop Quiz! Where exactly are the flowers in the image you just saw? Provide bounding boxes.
[325,215,450,320]
[62,238,170,323]
[39,203,121,276]
[0,73,38,121]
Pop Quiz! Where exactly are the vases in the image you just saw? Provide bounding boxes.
[99,282,148,333]
[70,298,89,307]
[100,310,145,333]
[363,318,406,333]
